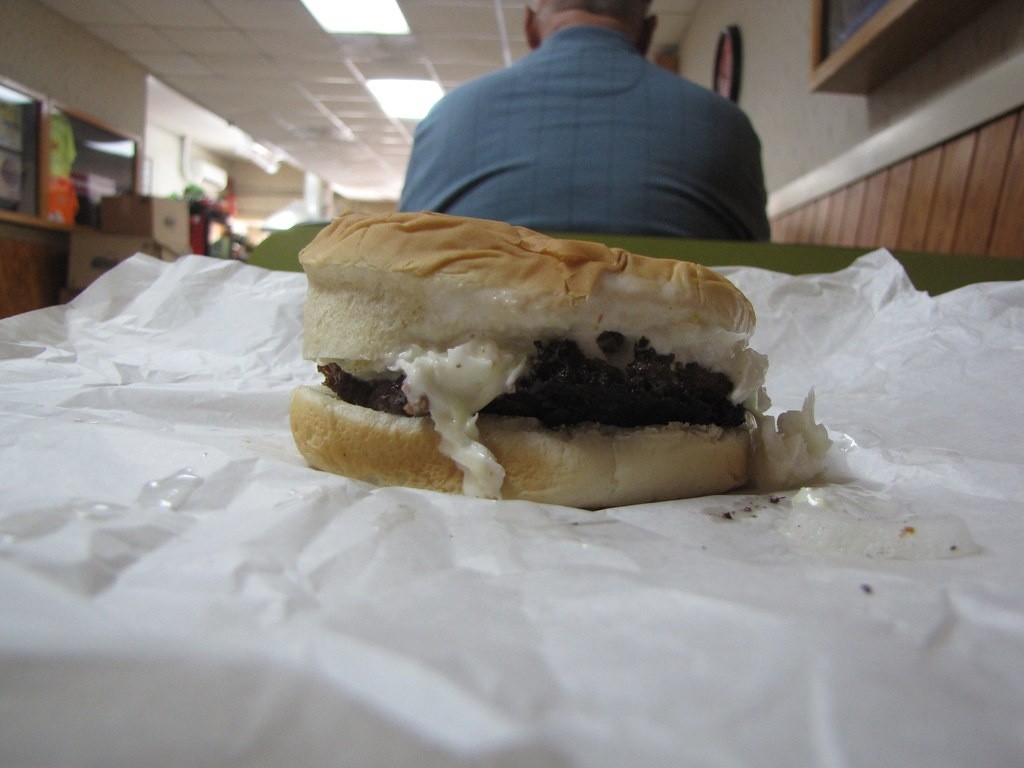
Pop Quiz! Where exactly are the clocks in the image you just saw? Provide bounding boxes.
[710,24,743,104]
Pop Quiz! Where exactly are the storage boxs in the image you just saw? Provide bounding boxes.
[102,196,190,262]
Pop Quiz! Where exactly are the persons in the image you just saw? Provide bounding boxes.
[399,1,770,243]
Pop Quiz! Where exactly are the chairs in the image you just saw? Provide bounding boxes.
[66,230,152,304]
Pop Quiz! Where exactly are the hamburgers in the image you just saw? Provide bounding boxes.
[288,209,772,510]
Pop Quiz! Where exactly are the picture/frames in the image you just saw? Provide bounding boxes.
[809,0,1004,96]
